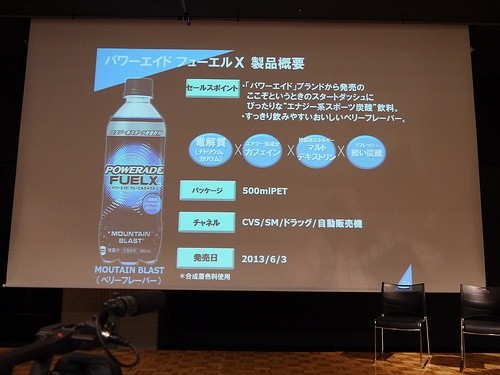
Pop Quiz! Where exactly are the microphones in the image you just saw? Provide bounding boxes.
[109,289,166,317]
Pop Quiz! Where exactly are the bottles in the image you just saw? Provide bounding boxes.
[99,78,167,265]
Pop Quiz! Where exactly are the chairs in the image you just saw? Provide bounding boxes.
[374,281,430,368]
[460,284,500,371]
